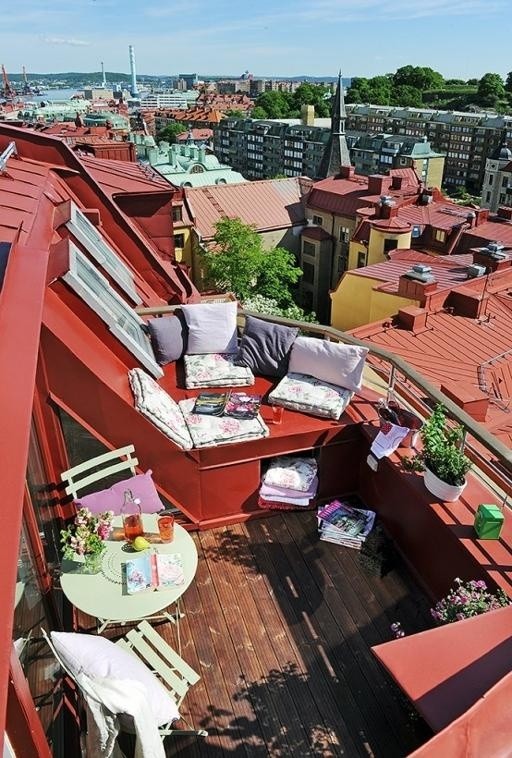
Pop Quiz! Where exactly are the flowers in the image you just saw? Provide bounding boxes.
[430,576,512,625]
[59,506,114,564]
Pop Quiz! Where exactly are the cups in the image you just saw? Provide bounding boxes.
[272,401,284,425]
[156,511,175,544]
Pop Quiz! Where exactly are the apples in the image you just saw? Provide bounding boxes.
[379,400,406,427]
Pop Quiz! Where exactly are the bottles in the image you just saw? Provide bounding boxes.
[377,387,402,426]
[120,488,145,543]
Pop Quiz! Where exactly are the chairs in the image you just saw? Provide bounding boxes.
[60,445,139,499]
[42,619,208,757]
[19,629,33,674]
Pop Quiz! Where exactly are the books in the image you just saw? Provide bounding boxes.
[316,497,376,551]
[126,550,185,596]
[190,389,262,419]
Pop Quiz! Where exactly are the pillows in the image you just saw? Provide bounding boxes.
[72,469,166,525]
[147,313,188,366]
[14,638,24,655]
[233,315,299,378]
[180,301,239,354]
[288,335,370,392]
[50,631,180,727]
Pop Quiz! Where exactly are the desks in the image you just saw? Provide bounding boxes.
[59,513,199,634]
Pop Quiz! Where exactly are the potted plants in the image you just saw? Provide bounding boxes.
[401,401,470,502]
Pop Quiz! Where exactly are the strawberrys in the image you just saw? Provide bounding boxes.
[378,422,392,434]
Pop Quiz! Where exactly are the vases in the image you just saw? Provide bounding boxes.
[85,556,103,575]
